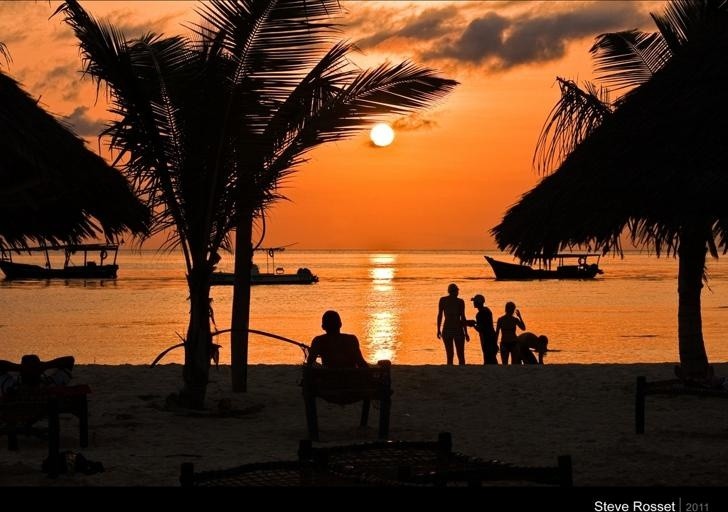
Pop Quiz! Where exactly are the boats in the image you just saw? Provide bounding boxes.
[205,242,319,288]
[0,237,119,286]
[482,246,605,284]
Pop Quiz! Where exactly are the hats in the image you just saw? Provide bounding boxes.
[471,294,485,302]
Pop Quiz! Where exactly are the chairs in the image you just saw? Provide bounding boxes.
[301,358,391,442]
[0,370,87,452]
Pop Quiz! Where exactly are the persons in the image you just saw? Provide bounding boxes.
[436,283,548,365]
[0,355,75,395]
[302,311,393,405]
